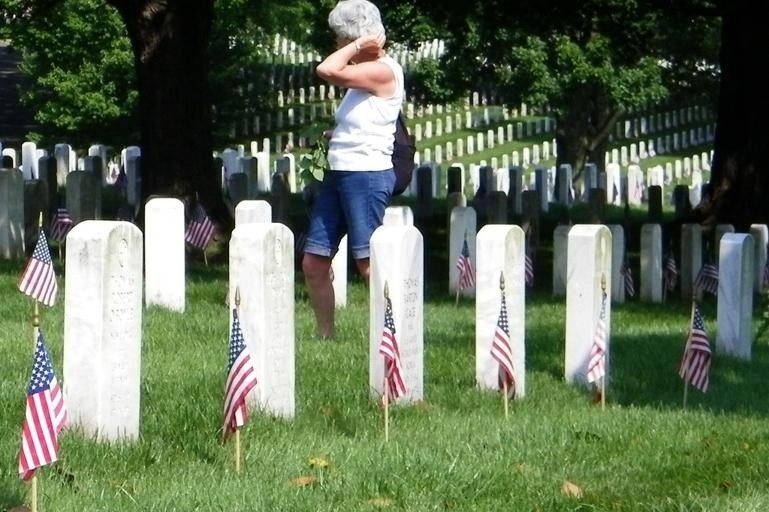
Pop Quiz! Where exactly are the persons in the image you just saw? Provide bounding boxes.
[300,1,405,341]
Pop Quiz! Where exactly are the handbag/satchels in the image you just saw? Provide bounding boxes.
[392,110,416,196]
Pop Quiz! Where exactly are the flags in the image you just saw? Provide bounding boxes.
[663,247,678,288]
[679,304,712,396]
[585,293,610,387]
[18,331,68,482]
[380,301,407,407]
[618,247,635,296]
[490,294,517,405]
[693,247,718,296]
[222,312,259,445]
[525,234,535,286]
[456,238,475,290]
[6,176,217,310]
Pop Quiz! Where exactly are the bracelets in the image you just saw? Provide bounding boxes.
[354,40,361,54]
